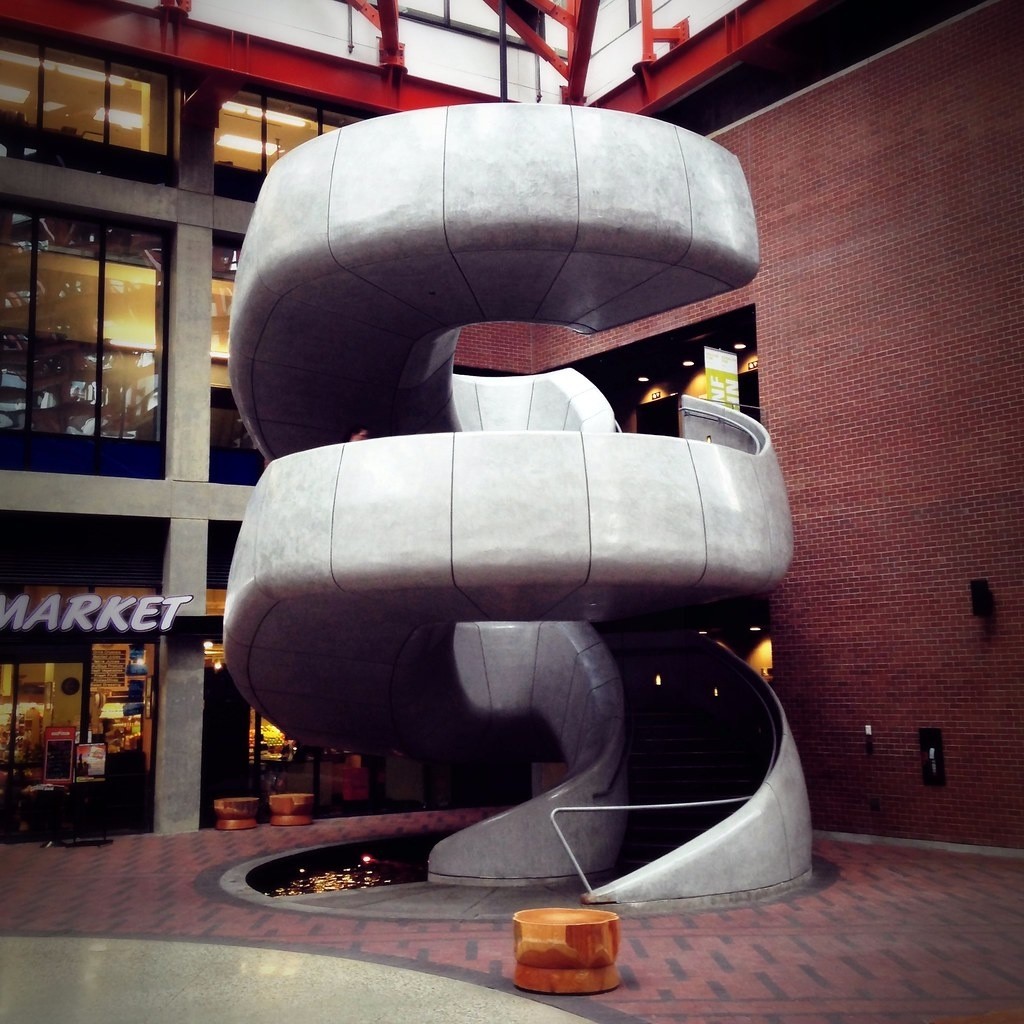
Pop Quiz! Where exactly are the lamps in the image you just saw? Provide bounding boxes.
[970,579,993,616]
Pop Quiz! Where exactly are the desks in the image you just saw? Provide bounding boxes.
[252,753,317,792]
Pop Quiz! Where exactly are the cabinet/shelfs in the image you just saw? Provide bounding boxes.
[0,707,39,758]
